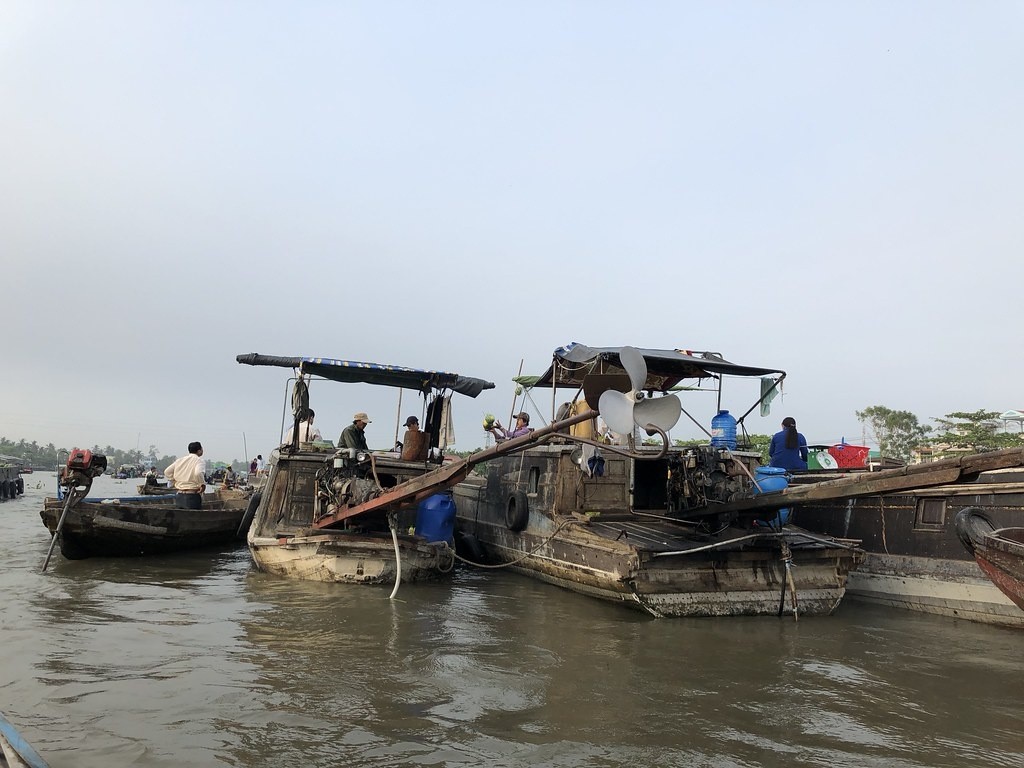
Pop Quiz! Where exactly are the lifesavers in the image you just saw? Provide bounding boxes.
[10,482,16,499]
[3,481,9,498]
[236,493,262,539]
[953,506,1003,558]
[0,481,4,500]
[459,533,485,560]
[18,478,24,493]
[504,489,529,532]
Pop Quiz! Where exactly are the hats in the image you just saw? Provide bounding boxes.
[402,416,418,426]
[150,466,156,470]
[353,412,373,424]
[513,412,530,421]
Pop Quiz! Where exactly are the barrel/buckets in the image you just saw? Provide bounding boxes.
[415,489,457,547]
[752,466,790,527]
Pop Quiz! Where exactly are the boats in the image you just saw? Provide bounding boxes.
[444,341,867,621]
[40,486,256,558]
[0,454,33,502]
[975,526,1024,612]
[247,439,463,587]
[111,467,269,496]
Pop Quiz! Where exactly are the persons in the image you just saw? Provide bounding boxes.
[164,443,206,509]
[251,455,265,473]
[485,412,531,442]
[215,466,237,484]
[397,416,423,448]
[769,417,808,470]
[338,413,372,449]
[145,467,158,487]
[284,409,323,448]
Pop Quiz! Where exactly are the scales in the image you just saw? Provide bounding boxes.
[800,444,838,469]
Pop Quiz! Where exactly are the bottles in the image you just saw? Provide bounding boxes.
[711,410,738,451]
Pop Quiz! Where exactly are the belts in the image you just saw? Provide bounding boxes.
[177,490,198,494]
